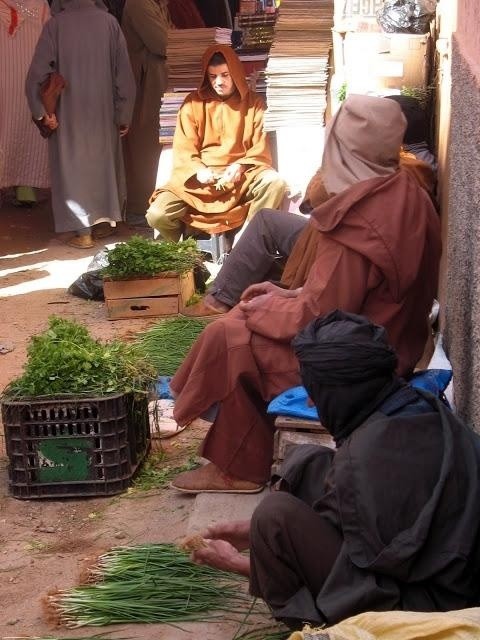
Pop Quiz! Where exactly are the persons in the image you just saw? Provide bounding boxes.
[144,44,287,248]
[171,93,442,494]
[178,95,438,321]
[189,309,480,631]
[24,1,171,249]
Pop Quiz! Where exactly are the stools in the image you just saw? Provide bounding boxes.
[183,221,228,262]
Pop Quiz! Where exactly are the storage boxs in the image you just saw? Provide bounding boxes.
[341,31,432,98]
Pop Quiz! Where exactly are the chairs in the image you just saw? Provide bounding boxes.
[270,298,441,488]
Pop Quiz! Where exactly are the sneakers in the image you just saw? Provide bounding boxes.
[93,223,113,239]
[126,211,149,227]
[182,293,229,316]
[67,237,94,249]
[170,463,264,493]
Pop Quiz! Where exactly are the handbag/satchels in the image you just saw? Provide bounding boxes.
[32,74,65,137]
